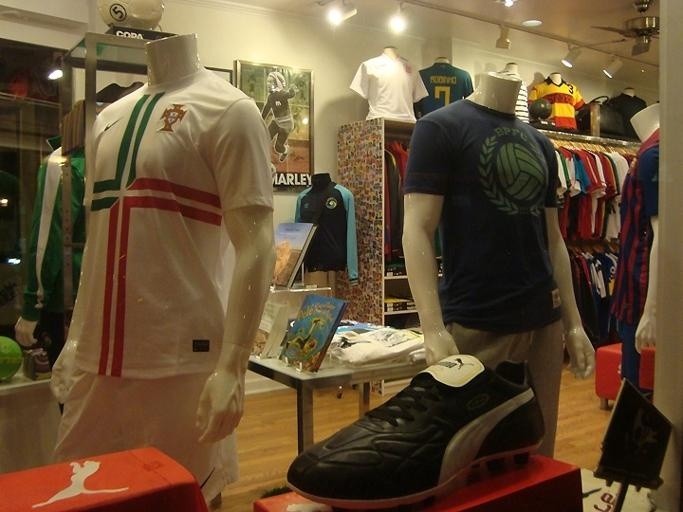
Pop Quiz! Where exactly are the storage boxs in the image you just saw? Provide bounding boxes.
[254,444,582,511]
[1,445,211,512]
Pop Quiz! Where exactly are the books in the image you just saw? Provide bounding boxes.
[278,294,348,372]
[272,222,318,287]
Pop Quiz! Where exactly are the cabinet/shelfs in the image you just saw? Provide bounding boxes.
[337,118,642,400]
[60,32,149,347]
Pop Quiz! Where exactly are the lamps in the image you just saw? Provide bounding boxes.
[561,40,625,79]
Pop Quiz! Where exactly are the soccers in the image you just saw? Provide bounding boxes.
[530,98,551,120]
[0,336,23,383]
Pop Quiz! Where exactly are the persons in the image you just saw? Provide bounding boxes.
[606,89,647,142]
[417,56,476,117]
[262,71,296,162]
[13,122,87,413]
[608,103,659,401]
[528,73,585,132]
[51,32,274,506]
[349,44,429,121]
[399,70,597,457]
[503,62,529,123]
[295,174,360,295]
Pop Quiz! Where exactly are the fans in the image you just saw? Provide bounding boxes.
[590,0,660,56]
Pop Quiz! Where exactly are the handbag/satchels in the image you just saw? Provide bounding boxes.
[577,101,623,131]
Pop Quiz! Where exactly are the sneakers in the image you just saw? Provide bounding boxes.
[285,355,544,508]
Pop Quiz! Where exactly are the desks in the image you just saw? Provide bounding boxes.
[247,318,428,455]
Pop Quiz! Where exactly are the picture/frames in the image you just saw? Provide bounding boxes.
[235,59,314,195]
